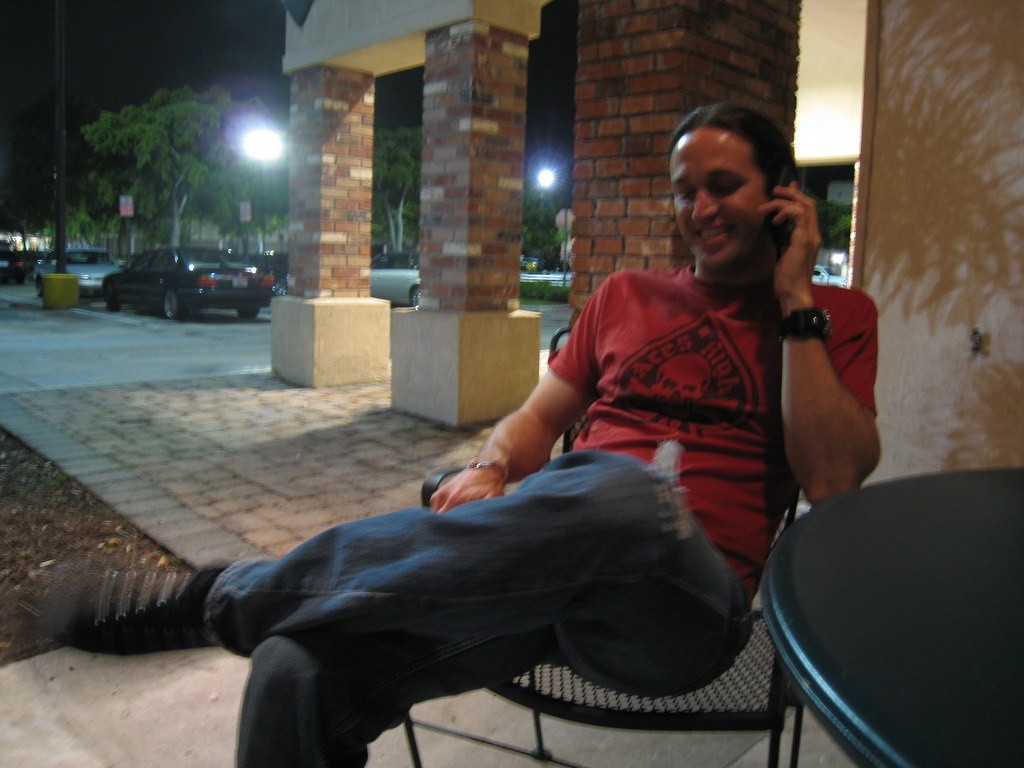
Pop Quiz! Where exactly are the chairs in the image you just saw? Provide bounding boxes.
[403,328,804,768]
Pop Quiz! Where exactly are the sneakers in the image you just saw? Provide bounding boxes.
[37,561,224,657]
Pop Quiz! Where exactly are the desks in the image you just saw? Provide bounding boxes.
[759,465,1024,768]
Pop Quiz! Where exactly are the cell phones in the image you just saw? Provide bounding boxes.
[762,170,792,262]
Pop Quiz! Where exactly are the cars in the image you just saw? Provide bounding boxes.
[249,250,289,296]
[0,242,28,285]
[370,250,420,309]
[33,243,124,299]
[101,244,277,321]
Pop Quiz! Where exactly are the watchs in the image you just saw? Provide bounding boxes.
[778,308,829,341]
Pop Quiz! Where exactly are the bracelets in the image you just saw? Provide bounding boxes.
[468,460,504,477]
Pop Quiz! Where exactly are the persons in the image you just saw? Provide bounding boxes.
[53,105,879,767]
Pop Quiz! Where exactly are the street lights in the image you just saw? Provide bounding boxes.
[245,128,283,254]
[537,168,568,285]
